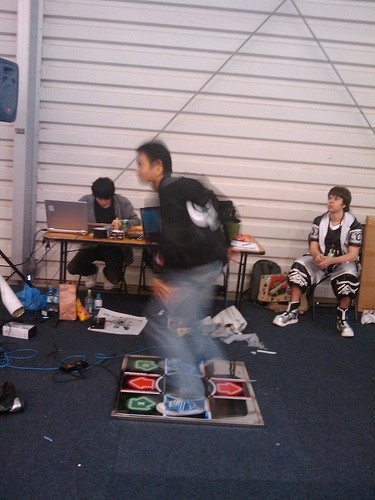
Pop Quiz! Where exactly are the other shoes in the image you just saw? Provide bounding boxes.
[103,273,114,292]
[155,396,206,416]
[84,266,100,288]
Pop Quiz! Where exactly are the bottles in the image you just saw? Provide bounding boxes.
[46,288,54,311]
[52,288,59,312]
[121,218,129,232]
[93,293,103,311]
[84,290,94,313]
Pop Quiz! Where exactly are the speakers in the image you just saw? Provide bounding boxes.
[0,58,19,122]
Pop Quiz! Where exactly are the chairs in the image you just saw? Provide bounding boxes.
[308,274,359,322]
[75,249,230,309]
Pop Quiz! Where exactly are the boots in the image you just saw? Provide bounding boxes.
[336,305,355,338]
[272,301,301,328]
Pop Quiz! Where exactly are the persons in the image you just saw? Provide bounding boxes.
[67,177,141,290]
[273,186,363,337]
[135,142,233,416]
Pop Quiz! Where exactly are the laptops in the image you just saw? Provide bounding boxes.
[45,200,92,235]
[139,206,161,243]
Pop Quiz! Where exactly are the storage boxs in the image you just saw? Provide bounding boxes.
[3,322,37,339]
[357,215,375,312]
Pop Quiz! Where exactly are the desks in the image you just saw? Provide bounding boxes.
[43,230,266,311]
